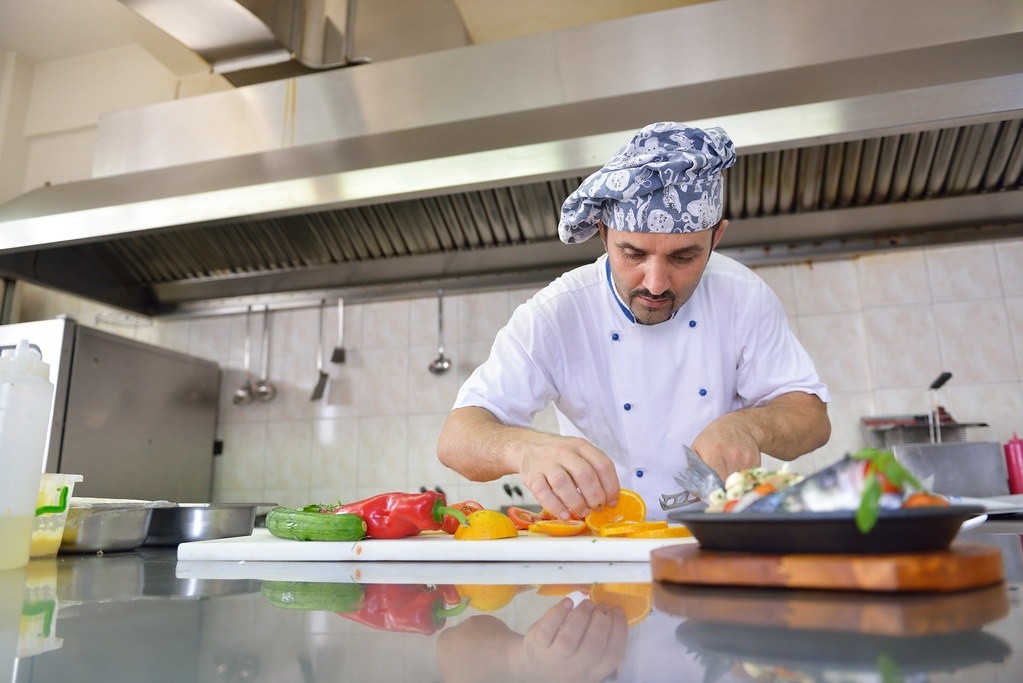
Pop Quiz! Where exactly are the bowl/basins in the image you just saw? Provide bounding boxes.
[31,472,85,559]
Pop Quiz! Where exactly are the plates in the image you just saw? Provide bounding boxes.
[666,503,987,552]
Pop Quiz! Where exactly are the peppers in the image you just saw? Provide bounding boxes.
[329,490,471,539]
[336,584,472,636]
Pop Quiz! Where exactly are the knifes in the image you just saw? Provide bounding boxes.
[657,492,702,510]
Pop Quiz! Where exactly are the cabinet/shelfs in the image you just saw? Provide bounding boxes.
[0,320,220,503]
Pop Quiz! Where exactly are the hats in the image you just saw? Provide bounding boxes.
[557,122,737,245]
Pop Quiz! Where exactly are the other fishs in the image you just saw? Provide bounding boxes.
[674,444,903,514]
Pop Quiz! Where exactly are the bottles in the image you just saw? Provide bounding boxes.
[0,339,55,571]
[1003,430,1023,495]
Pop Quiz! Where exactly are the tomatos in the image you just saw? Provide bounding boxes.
[441,501,542,536]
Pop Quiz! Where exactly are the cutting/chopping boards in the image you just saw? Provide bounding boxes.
[176,528,697,564]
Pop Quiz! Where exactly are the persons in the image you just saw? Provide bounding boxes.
[435,582,884,683]
[436,119,833,528]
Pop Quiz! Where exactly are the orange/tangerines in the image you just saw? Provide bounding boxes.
[454,489,694,539]
[455,584,654,627]
